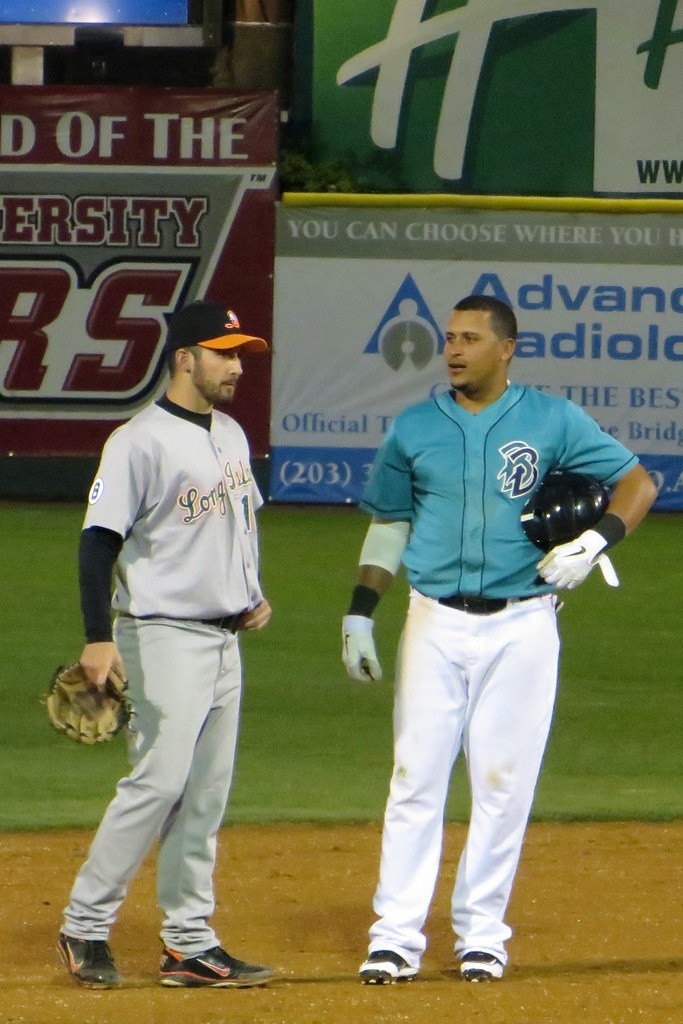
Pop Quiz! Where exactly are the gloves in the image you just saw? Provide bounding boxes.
[536,530,619,590]
[342,615,382,683]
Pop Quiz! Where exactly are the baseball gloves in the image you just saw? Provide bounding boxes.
[44,663,137,746]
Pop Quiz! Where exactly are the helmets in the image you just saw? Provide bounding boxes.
[521,472,609,554]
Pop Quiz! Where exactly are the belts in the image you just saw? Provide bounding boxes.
[117,609,246,635]
[439,593,533,615]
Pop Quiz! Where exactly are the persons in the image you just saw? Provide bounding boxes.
[343,294,656,982]
[57,301,272,989]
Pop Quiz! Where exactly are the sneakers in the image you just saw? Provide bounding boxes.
[358,950,418,985]
[159,945,273,987]
[56,932,121,990]
[460,951,503,983]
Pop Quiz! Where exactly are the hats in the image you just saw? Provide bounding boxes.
[164,300,268,353]
